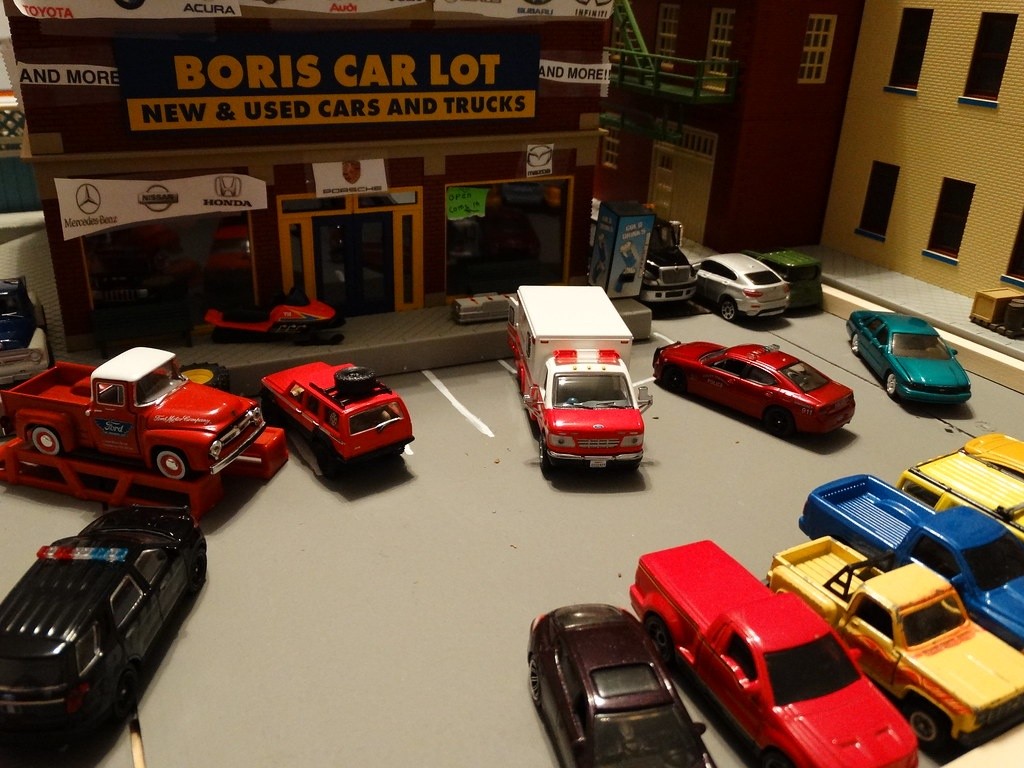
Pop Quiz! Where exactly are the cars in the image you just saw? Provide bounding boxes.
[0,276,55,385]
[653,341,856,438]
[696,248,822,320]
[964,432,1024,479]
[846,311,971,407]
[526,604,717,768]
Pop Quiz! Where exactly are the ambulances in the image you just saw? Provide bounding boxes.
[507,286,657,482]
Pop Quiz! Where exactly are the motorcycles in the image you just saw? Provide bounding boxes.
[204,284,347,339]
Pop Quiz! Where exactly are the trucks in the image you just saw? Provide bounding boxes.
[591,197,698,302]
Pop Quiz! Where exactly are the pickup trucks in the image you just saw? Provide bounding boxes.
[0,346,266,482]
[630,540,919,768]
[767,536,1024,755]
[797,474,1024,650]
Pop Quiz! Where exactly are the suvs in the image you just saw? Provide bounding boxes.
[0,508,207,768]
[896,449,1024,542]
[260,360,415,478]
